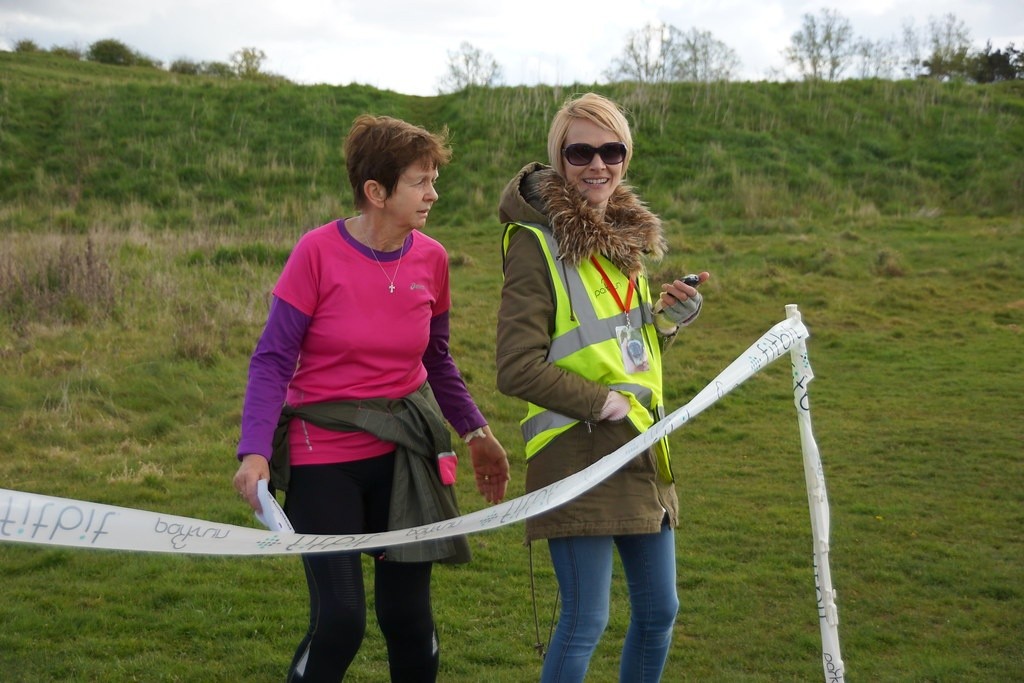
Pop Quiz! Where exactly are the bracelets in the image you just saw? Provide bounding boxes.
[464,428,487,443]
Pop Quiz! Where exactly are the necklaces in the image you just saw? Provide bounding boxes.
[358,215,404,293]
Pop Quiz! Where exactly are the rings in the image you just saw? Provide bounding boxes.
[485,476,489,480]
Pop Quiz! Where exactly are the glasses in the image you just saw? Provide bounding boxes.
[562,141,628,166]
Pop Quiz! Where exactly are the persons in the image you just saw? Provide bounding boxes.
[497,93,710,683]
[234,120,510,683]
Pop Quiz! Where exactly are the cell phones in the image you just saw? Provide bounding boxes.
[657,275,700,314]
